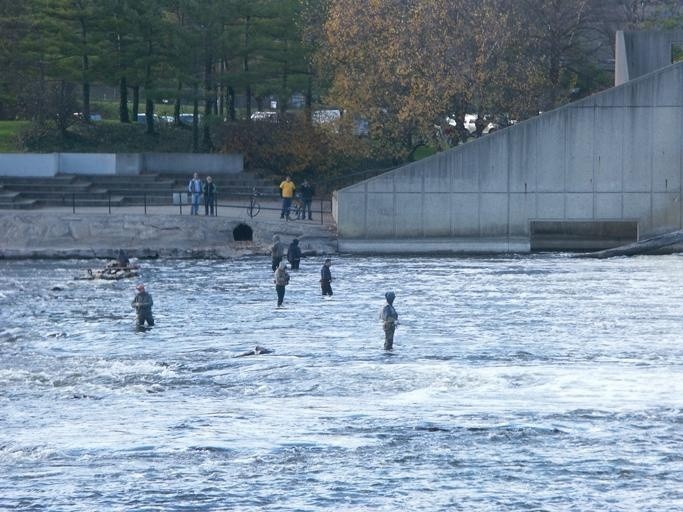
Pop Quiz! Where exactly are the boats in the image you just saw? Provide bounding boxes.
[74,267,94,280]
[100,262,140,280]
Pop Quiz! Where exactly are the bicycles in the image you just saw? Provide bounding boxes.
[247,186,263,217]
[286,192,305,221]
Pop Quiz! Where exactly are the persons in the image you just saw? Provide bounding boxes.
[279,176,296,220]
[131,284,154,326]
[266,235,284,272]
[301,178,313,221]
[202,176,216,216]
[319,259,333,297]
[379,291,400,351]
[286,239,301,270]
[275,261,288,306]
[189,172,203,215]
